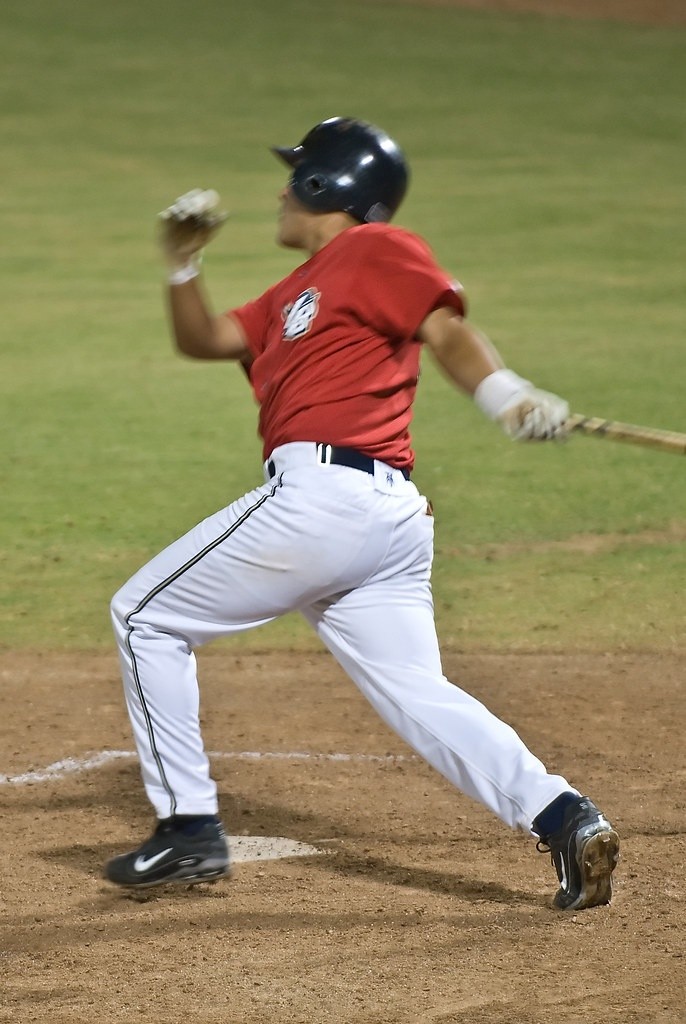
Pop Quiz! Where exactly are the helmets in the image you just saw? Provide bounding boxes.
[270,115,409,224]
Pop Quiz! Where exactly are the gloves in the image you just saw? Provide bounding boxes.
[473,369,571,445]
[157,189,224,287]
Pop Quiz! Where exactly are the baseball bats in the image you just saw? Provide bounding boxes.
[518,405,686,457]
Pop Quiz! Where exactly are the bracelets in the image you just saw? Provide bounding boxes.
[474,369,534,419]
[167,263,200,285]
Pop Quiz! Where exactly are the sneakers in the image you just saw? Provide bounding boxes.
[531,797,620,910]
[103,817,232,889]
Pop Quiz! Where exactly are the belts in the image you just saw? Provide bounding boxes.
[269,443,412,481]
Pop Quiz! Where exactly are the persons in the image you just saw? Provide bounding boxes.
[103,115,622,908]
